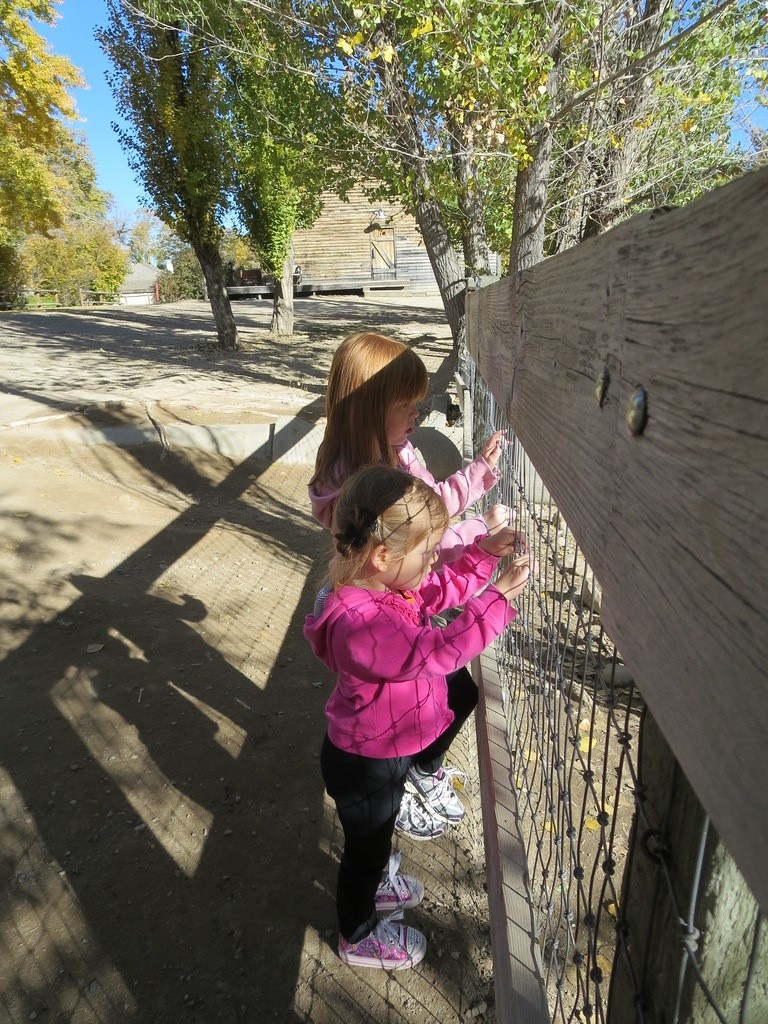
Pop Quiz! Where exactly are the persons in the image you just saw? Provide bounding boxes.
[307,331,513,842]
[301,463,539,972]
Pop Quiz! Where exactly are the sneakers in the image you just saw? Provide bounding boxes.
[374,851,425,910]
[394,791,449,842]
[403,759,468,824]
[338,910,426,970]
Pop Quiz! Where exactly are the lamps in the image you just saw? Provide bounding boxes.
[373,209,387,219]
